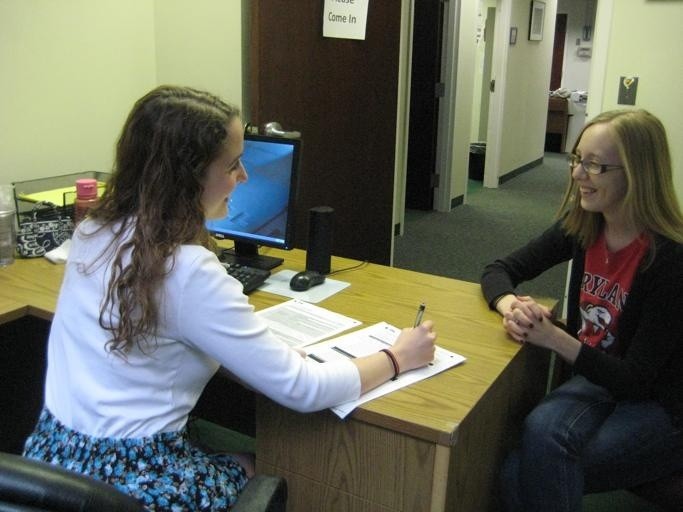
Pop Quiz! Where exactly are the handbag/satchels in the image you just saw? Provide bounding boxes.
[18,200,74,258]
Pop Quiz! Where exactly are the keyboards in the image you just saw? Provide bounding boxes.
[221,263,270,295]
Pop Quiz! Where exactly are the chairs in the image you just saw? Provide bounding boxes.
[1,448,287,511]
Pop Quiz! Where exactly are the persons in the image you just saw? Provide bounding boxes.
[480,107,682,511]
[20,83,438,512]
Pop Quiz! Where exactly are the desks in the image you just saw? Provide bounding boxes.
[1,240,559,511]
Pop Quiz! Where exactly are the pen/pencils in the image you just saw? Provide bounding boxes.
[414,304,425,326]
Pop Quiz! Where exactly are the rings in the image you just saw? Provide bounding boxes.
[515,319,519,324]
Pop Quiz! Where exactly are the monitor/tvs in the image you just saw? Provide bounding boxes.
[203,133,301,269]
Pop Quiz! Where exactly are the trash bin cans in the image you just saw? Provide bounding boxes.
[469,143,486,180]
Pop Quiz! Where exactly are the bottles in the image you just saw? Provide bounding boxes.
[1,208,16,265]
[72,178,100,228]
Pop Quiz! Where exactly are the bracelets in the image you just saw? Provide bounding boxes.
[493,294,505,310]
[378,348,399,379]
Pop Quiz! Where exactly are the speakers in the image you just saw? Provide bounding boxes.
[305,205,334,276]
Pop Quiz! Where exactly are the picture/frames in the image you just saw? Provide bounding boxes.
[510,28,518,44]
[529,0,546,42]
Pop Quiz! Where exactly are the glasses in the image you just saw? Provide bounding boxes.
[566,154,624,175]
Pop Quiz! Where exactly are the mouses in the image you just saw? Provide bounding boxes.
[290,267,325,293]
